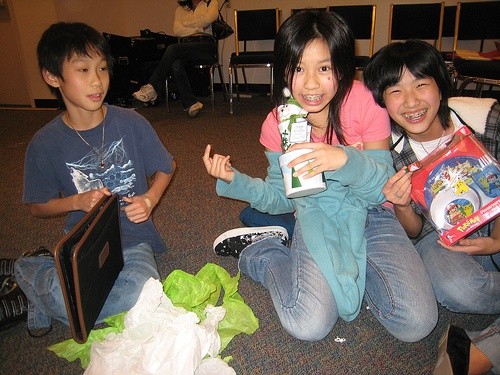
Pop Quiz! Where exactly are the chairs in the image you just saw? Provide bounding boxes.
[165,0,500,114]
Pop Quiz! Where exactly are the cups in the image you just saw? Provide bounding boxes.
[280,148,328,198]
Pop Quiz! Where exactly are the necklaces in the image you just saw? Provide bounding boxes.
[65,106,106,169]
[419,129,444,155]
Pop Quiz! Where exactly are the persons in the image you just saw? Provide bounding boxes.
[201,10,440,342]
[0,21,178,336]
[360,38,500,375]
[132,0,219,116]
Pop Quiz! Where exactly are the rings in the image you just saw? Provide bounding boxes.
[395,193,401,198]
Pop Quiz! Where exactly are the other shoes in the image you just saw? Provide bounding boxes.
[434,323,472,375]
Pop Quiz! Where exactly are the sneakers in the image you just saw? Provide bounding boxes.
[0,291,30,329]
[0,245,53,298]
[132,84,157,102]
[189,100,203,117]
[213,226,289,259]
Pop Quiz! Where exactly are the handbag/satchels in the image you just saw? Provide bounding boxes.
[207,0,234,40]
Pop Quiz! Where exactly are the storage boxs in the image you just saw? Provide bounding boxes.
[396,126,500,246]
